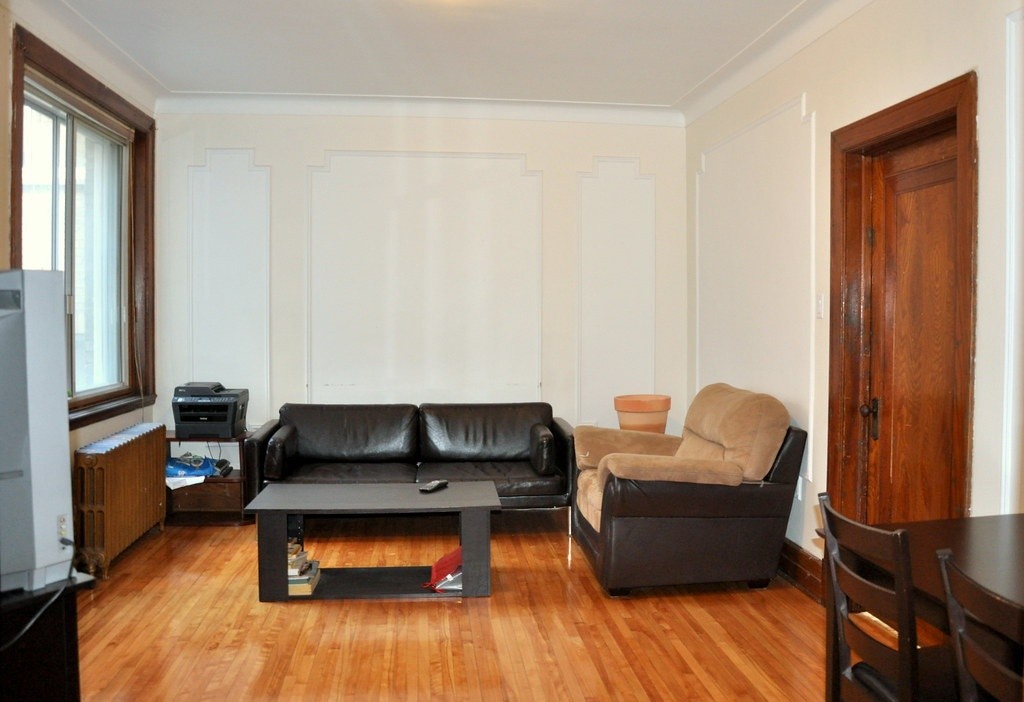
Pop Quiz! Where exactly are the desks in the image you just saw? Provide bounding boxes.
[0,572,95,702]
[816,513,1024,673]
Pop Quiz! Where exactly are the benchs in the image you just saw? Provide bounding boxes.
[243,403,576,537]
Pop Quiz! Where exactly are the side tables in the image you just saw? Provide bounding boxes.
[167,429,253,527]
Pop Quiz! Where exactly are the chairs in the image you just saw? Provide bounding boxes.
[818,491,993,702]
[934,546,1024,702]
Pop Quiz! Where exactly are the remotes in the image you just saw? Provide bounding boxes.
[419,480,448,493]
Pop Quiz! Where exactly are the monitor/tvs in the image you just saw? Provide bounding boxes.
[0,267,77,592]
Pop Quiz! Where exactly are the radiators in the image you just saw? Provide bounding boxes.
[72,421,167,581]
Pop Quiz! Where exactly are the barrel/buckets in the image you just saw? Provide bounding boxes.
[614,394,671,434]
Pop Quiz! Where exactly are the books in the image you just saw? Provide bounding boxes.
[287,537,321,596]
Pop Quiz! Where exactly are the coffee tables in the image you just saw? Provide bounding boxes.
[244,480,502,602]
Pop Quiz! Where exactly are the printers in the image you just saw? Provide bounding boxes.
[172,381,249,440]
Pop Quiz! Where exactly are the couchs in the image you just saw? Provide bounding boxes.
[572,382,808,599]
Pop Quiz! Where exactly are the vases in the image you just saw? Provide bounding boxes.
[613,393,672,434]
